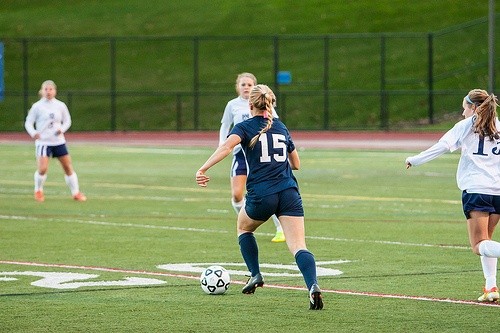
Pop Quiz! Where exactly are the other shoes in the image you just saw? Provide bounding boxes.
[73,192,86,201]
[35,191,45,201]
[271,230,285,242]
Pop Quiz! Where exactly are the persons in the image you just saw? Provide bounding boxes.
[405,89,500,303]
[196,72,324,311]
[24,79,87,202]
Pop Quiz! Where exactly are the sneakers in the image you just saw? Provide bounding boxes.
[242,273,264,294]
[309,284,323,310]
[477,286,499,302]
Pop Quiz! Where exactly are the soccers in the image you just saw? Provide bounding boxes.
[200,265,231,296]
